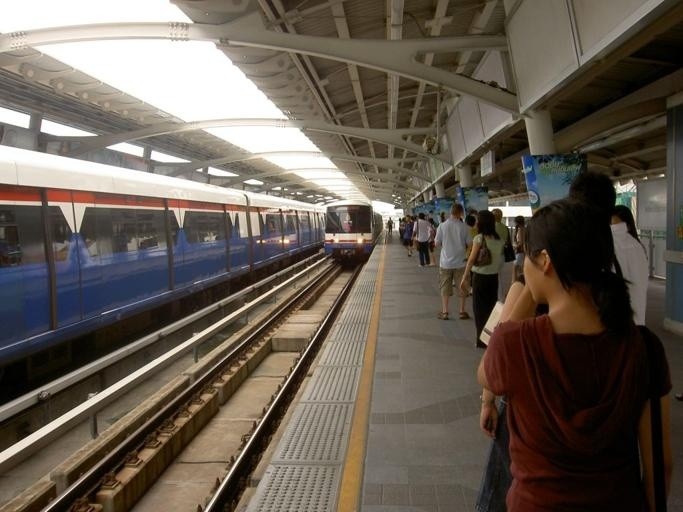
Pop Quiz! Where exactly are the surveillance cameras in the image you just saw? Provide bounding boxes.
[423,137,436,152]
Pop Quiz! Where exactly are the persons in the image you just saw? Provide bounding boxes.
[434,203,528,348]
[399,213,436,267]
[475,172,672,511]
[388,218,393,232]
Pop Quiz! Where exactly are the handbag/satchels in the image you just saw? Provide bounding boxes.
[479,302,504,346]
[413,240,419,251]
[474,248,490,265]
[505,246,516,262]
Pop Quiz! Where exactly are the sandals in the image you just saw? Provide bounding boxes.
[438,312,448,320]
[460,312,469,319]
